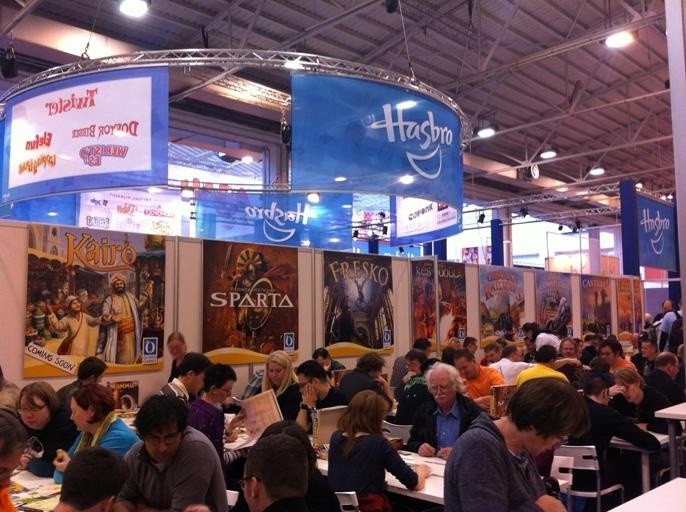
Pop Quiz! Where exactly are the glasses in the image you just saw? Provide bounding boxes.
[17,403,47,415]
[428,382,453,394]
[559,436,568,445]
[0,461,24,479]
[146,431,180,445]
[299,378,312,387]
[238,474,262,490]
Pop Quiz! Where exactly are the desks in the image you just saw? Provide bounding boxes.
[610,430,670,494]
[11,470,59,512]
[607,476,686,512]
[655,402,686,481]
[316,439,446,507]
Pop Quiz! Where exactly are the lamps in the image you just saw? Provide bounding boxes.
[477,31,673,202]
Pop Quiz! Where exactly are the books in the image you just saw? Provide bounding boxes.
[222,389,284,453]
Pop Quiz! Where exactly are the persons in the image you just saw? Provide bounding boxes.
[241,299,685,512]
[157,351,212,405]
[102,274,153,365]
[17,382,78,477]
[52,386,141,484]
[461,244,492,266]
[185,363,248,487]
[369,211,387,236]
[1,364,21,417]
[47,296,109,359]
[49,446,130,512]
[56,357,108,445]
[114,395,229,511]
[165,332,189,381]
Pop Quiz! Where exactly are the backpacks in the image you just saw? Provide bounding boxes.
[668,310,686,354]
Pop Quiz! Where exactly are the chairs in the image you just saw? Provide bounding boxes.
[548,456,576,512]
[226,489,239,512]
[383,420,412,444]
[549,443,624,512]
[332,492,361,512]
